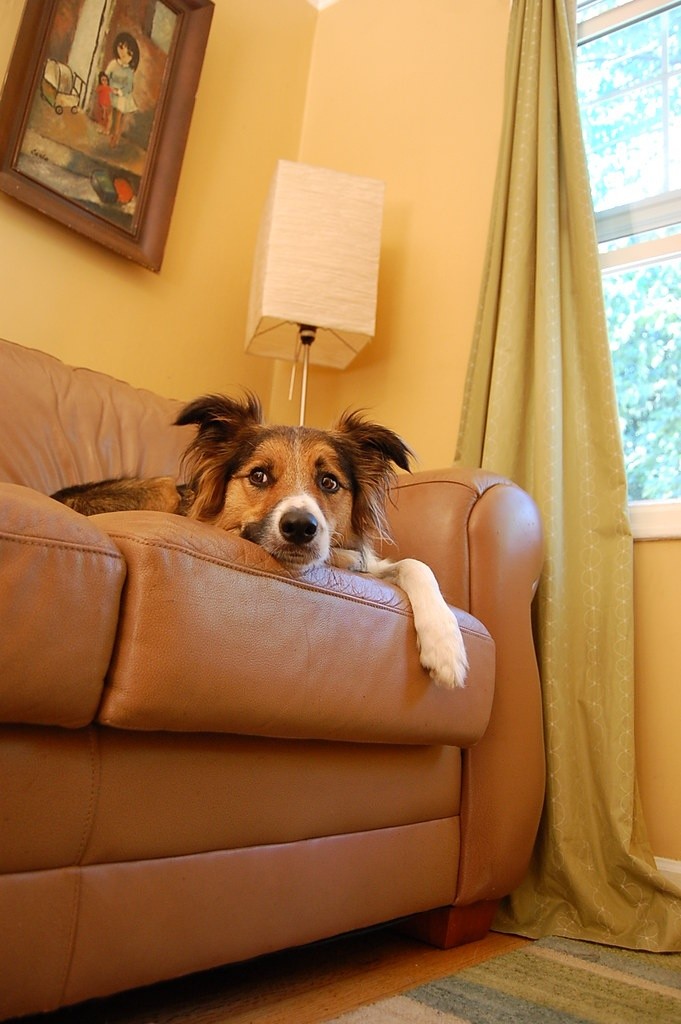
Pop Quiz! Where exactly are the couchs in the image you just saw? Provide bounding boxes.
[0,339,547,1024]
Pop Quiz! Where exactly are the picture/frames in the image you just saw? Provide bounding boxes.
[0,0,215,274]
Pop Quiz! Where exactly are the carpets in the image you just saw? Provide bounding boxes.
[319,936,680,1024]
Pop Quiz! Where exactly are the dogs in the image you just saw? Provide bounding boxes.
[48,388,470,687]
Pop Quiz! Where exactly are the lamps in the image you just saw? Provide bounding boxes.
[245,160,386,427]
